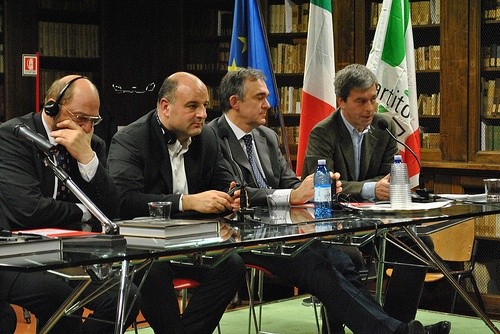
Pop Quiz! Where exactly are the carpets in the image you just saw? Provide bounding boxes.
[121,293,500,334]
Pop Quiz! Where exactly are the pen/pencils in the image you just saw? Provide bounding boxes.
[229,184,239,195]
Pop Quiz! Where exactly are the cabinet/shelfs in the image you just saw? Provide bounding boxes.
[184,0,500,319]
[0,0,7,123]
[31,0,106,138]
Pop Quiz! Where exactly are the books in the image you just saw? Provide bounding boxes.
[118,218,220,239]
[269,3,310,152]
[39,10,233,106]
[371,0,440,148]
[0,233,63,257]
[481,0,500,153]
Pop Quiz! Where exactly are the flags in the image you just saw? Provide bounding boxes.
[365,0,421,190]
[295,0,335,180]
[227,0,276,109]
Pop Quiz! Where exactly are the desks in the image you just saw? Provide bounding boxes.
[0,196,500,334]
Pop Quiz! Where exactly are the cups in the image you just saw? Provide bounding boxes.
[147,201,173,220]
[484,179,500,203]
[390,162,413,208]
[267,194,288,220]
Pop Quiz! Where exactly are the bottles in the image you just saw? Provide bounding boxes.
[393,155,402,163]
[313,158,332,218]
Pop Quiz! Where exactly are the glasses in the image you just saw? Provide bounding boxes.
[62,105,102,125]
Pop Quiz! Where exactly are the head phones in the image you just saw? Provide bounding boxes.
[155,111,177,145]
[44,75,88,117]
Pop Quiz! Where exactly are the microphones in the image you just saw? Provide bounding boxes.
[6,117,56,154]
[217,126,261,225]
[377,119,435,202]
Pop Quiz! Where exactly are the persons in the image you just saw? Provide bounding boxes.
[0,75,142,334]
[107,72,246,334]
[208,64,451,334]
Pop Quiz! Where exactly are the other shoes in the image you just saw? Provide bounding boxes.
[423,321,451,334]
[393,320,426,334]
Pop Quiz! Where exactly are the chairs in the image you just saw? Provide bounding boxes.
[135,217,484,334]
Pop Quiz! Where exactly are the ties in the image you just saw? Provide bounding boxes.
[56,145,73,200]
[243,134,270,189]
